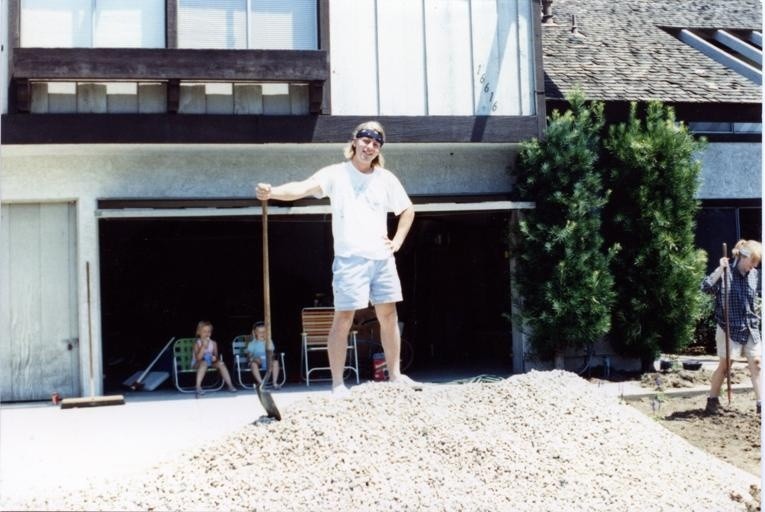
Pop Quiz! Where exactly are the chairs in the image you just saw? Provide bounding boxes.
[171,305,360,394]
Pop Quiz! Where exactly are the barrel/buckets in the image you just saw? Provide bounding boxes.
[371,352,389,381]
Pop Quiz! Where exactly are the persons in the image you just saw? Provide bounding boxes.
[243,321,281,390]
[700,239,761,416]
[190,321,237,395]
[255,120,424,390]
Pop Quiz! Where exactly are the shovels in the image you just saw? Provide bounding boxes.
[251,200,283,422]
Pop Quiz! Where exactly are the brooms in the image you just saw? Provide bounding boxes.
[59,259,125,409]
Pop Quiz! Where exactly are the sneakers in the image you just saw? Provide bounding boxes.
[756,400,761,412]
[396,373,422,391]
[706,397,722,414]
